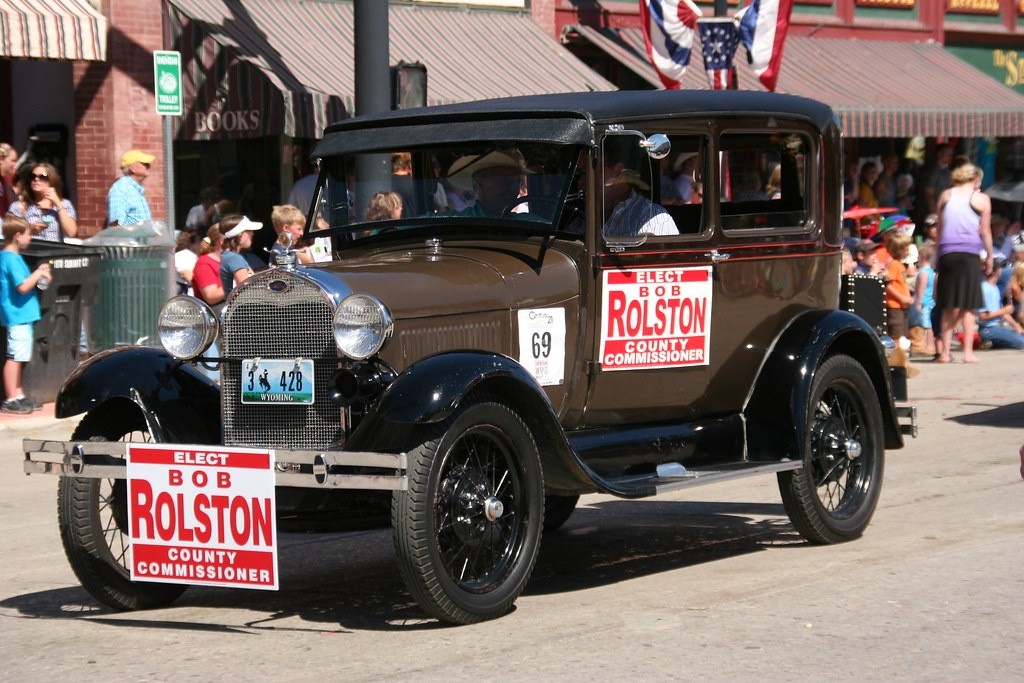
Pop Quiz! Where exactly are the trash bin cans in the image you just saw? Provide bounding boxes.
[84,233,175,355]
[0,238,105,403]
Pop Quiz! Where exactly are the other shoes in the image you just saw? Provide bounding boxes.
[978,338,993,351]
[933,351,953,361]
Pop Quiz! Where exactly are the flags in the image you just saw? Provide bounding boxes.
[638,0,795,95]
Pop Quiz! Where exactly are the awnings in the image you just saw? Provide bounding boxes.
[561,24,1023,138]
[166,0,619,141]
[0,2,109,64]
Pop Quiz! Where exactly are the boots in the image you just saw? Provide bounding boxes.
[909,325,927,356]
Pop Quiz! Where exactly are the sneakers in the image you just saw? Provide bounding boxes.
[1,399,32,414]
[17,397,43,410]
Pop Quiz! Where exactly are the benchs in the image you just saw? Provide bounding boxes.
[663,202,797,233]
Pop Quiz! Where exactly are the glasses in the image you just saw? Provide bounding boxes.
[27,173,49,183]
[568,160,601,183]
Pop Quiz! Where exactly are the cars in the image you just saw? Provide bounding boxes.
[21,91,918,626]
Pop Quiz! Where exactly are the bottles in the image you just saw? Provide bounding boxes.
[37,268,49,290]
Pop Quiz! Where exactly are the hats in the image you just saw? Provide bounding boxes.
[120,149,155,168]
[222,215,264,238]
[447,149,537,192]
[675,152,697,172]
[853,238,882,262]
[924,213,939,227]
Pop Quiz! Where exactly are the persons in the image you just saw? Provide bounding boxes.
[0,145,1024,413]
